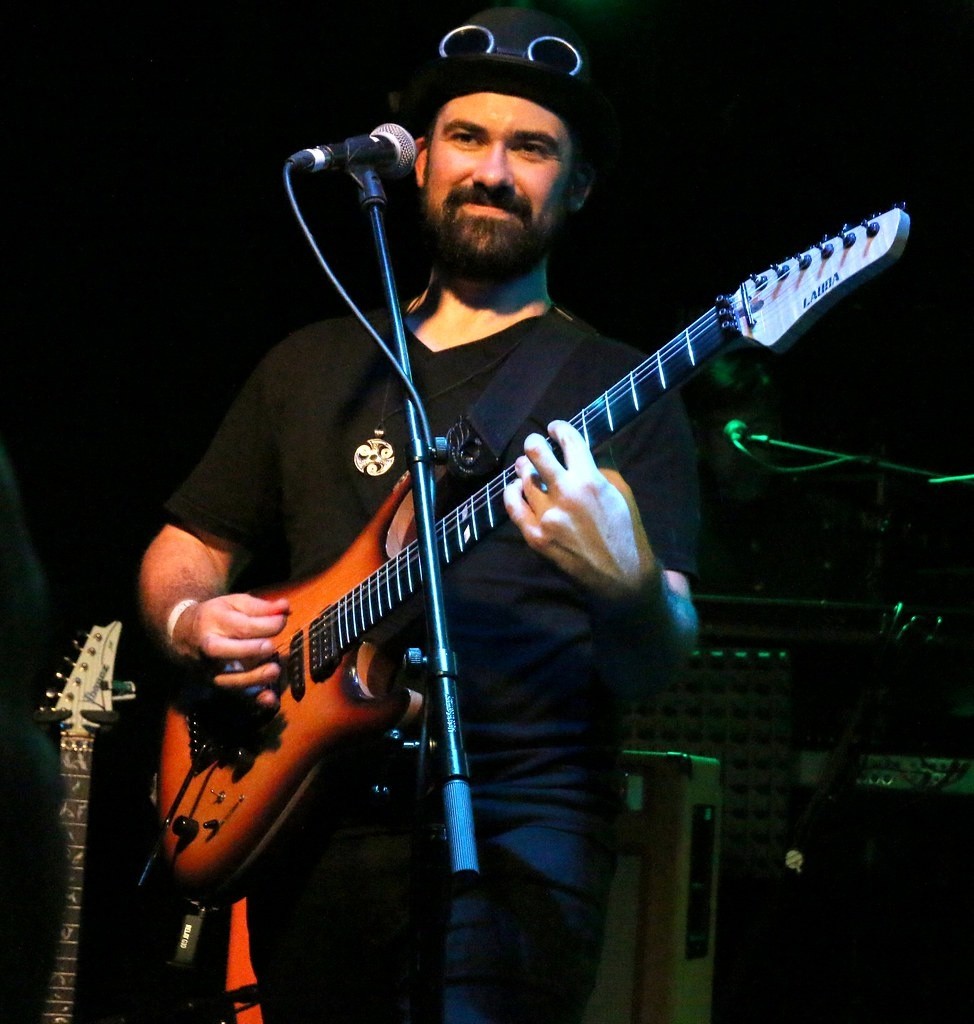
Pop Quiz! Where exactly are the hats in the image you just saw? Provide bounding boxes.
[398,6,619,166]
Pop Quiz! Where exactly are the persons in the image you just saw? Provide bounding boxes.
[137,5,707,1024]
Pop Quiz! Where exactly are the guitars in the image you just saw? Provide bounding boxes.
[152,193,917,922]
[35,615,139,1024]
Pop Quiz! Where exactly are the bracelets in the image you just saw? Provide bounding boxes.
[165,598,197,650]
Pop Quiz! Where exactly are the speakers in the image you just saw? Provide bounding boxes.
[583,750,723,1024]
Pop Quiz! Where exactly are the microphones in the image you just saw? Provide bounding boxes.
[722,419,749,444]
[286,123,416,180]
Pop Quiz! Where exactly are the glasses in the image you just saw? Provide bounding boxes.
[438,24,582,76]
